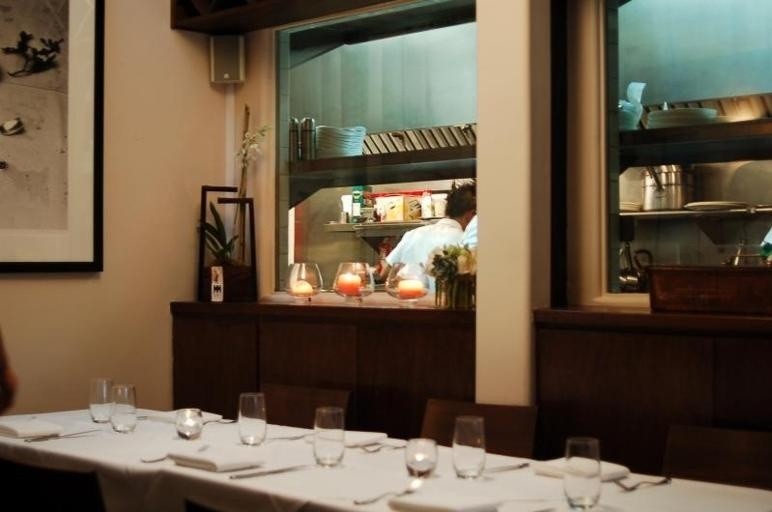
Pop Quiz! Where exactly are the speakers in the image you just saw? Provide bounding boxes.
[210,35,246,83]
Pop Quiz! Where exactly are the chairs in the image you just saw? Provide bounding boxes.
[659,424,772,490]
[255,378,352,429]
[419,397,540,459]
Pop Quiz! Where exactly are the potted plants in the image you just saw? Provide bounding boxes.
[199,204,238,300]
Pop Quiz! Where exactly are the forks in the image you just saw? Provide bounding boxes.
[353,473,427,505]
[360,444,409,453]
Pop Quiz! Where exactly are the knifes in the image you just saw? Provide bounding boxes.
[140,443,217,462]
[231,463,312,479]
[27,428,106,442]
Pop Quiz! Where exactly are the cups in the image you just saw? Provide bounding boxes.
[89,378,117,424]
[563,435,603,510]
[384,261,430,304]
[175,408,206,440]
[285,262,323,304]
[237,394,268,446]
[201,418,237,426]
[312,405,347,469]
[618,104,642,130]
[110,384,138,435]
[453,415,487,480]
[405,437,437,479]
[332,261,377,302]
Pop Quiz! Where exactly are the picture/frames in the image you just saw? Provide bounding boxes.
[0,0,103,273]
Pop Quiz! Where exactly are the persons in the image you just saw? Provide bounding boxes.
[345,184,477,295]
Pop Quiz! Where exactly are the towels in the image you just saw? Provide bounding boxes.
[166,445,269,473]
[145,409,223,426]
[306,430,388,449]
[3,414,60,439]
[530,454,630,482]
[388,479,503,511]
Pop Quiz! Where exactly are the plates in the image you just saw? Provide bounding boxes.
[644,106,718,129]
[619,201,638,212]
[315,125,367,156]
[685,199,749,211]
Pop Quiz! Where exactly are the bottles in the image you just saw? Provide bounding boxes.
[289,115,316,161]
[422,188,433,218]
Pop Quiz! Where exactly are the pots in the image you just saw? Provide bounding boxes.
[638,163,696,212]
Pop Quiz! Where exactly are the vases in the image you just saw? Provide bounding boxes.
[230,222,251,299]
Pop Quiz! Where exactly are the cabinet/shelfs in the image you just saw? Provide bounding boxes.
[289,114,476,233]
[618,116,772,233]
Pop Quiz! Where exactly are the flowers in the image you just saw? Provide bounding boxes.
[234,103,274,220]
